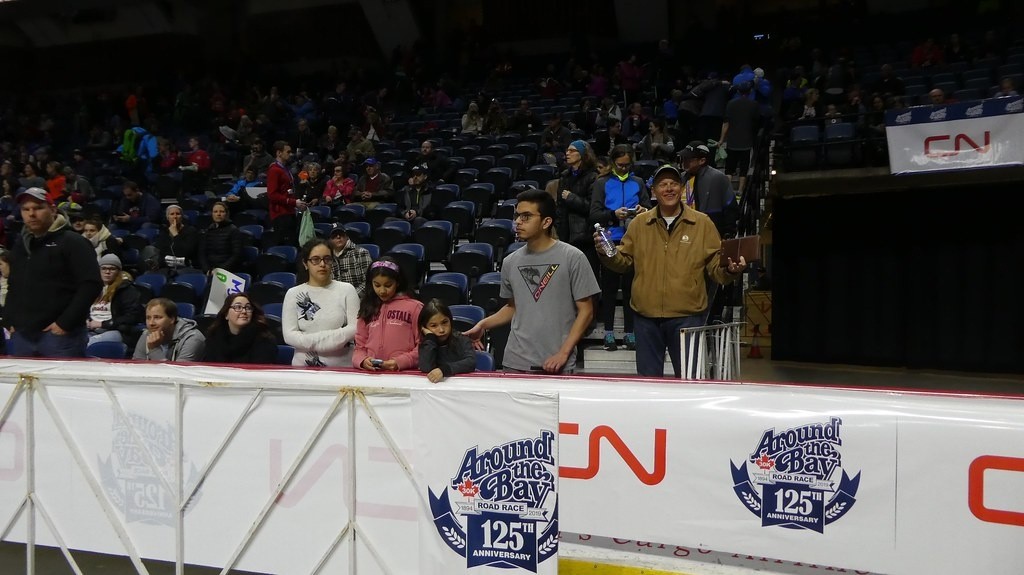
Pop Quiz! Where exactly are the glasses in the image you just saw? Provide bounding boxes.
[614,162,631,168]
[306,255,333,265]
[514,212,543,221]
[99,265,120,272]
[330,231,345,238]
[655,182,681,188]
[229,303,251,311]
[412,171,423,176]
[567,148,579,152]
[71,216,84,223]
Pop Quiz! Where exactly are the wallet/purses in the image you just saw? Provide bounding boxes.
[718,236,763,267]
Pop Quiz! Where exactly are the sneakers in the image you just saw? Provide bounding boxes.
[623,334,637,350]
[603,332,618,350]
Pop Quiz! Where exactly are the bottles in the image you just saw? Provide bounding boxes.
[226,196,240,202]
[294,195,306,210]
[594,223,618,257]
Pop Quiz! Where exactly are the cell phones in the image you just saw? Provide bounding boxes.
[628,209,636,215]
[370,359,383,367]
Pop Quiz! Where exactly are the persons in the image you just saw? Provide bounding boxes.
[0,30,1018,384]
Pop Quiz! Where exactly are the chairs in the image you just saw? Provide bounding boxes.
[0,38,1024,371]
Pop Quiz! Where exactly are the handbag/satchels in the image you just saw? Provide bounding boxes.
[707,136,727,163]
[298,206,318,251]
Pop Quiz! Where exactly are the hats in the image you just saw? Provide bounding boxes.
[327,222,346,237]
[247,164,260,174]
[754,67,764,77]
[738,82,752,95]
[349,126,361,133]
[359,158,377,166]
[412,161,428,174]
[680,140,710,160]
[653,164,682,181]
[16,186,52,202]
[98,253,123,271]
